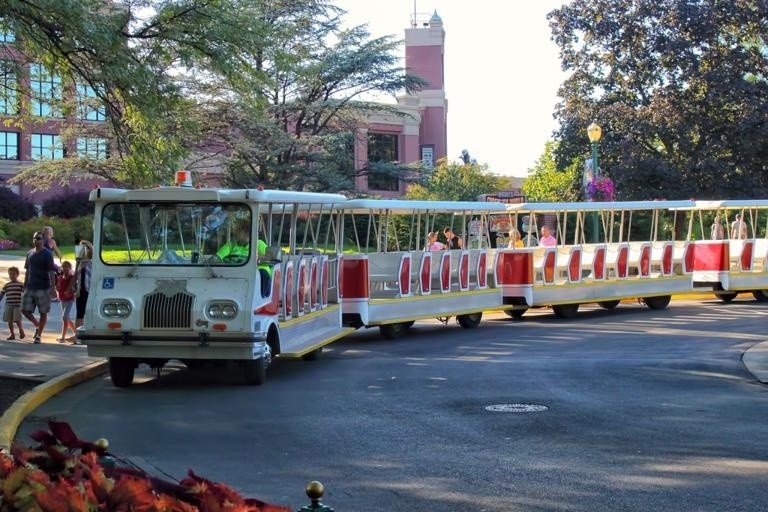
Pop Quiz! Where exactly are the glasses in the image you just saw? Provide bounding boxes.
[32,237,45,241]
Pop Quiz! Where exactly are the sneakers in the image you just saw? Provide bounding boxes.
[19,329,25,339]
[6,333,15,340]
[33,329,41,344]
[67,336,83,345]
[56,337,65,343]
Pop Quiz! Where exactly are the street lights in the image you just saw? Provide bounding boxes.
[588,122,603,244]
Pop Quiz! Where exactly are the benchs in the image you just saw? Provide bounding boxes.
[730,240,767,272]
[508,240,695,284]
[367,249,504,299]
[280,252,343,320]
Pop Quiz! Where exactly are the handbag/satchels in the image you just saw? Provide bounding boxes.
[74,245,88,261]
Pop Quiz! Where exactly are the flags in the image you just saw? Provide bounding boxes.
[582,156,593,202]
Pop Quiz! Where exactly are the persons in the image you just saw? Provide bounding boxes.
[423,230,445,251]
[522,230,538,247]
[710,217,725,240]
[0,225,93,345]
[539,225,556,246]
[508,229,522,248]
[209,215,273,298]
[439,226,462,250]
[731,214,748,239]
